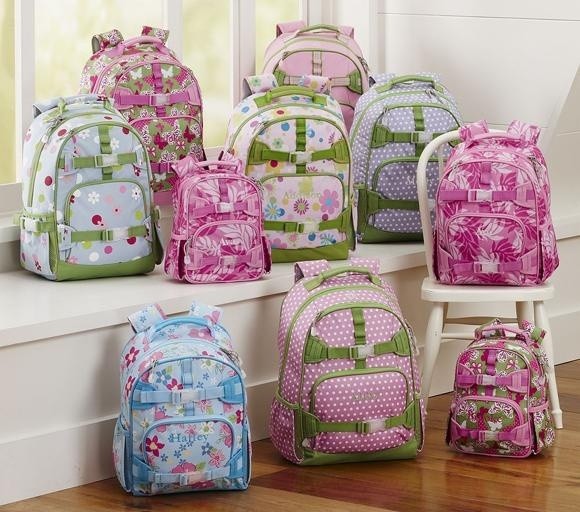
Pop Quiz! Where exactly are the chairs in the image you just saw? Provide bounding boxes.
[417,128,566,428]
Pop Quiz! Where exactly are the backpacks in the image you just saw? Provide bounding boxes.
[433,120,559,287]
[266,257,427,467]
[164,151,273,284]
[226,71,356,263]
[445,320,555,459]
[350,74,466,244]
[112,300,253,497]
[261,21,378,136]
[19,94,165,281]
[80,26,209,251]
